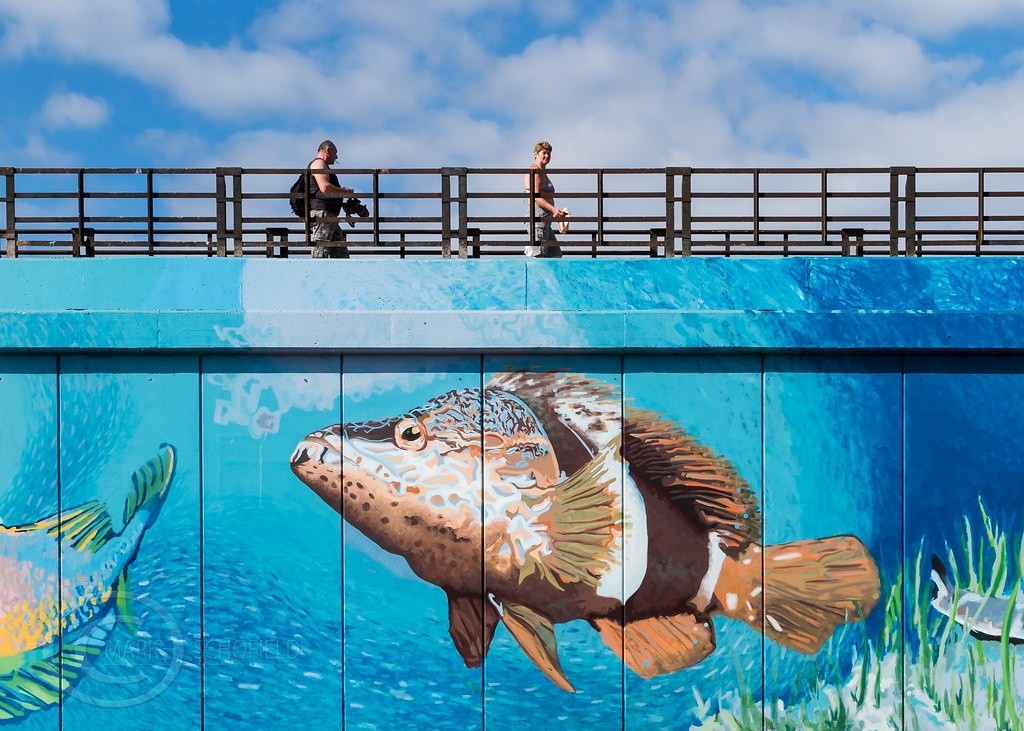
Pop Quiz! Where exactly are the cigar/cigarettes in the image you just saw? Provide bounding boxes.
[334,160,339,164]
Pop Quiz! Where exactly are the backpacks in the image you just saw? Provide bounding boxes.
[290,158,329,217]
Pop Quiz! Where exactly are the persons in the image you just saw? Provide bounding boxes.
[525,141,569,258]
[305,139,356,258]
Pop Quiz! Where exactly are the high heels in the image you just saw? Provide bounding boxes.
[561,207,570,232]
[557,210,563,233]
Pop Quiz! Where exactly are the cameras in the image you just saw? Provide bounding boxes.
[345,199,369,218]
